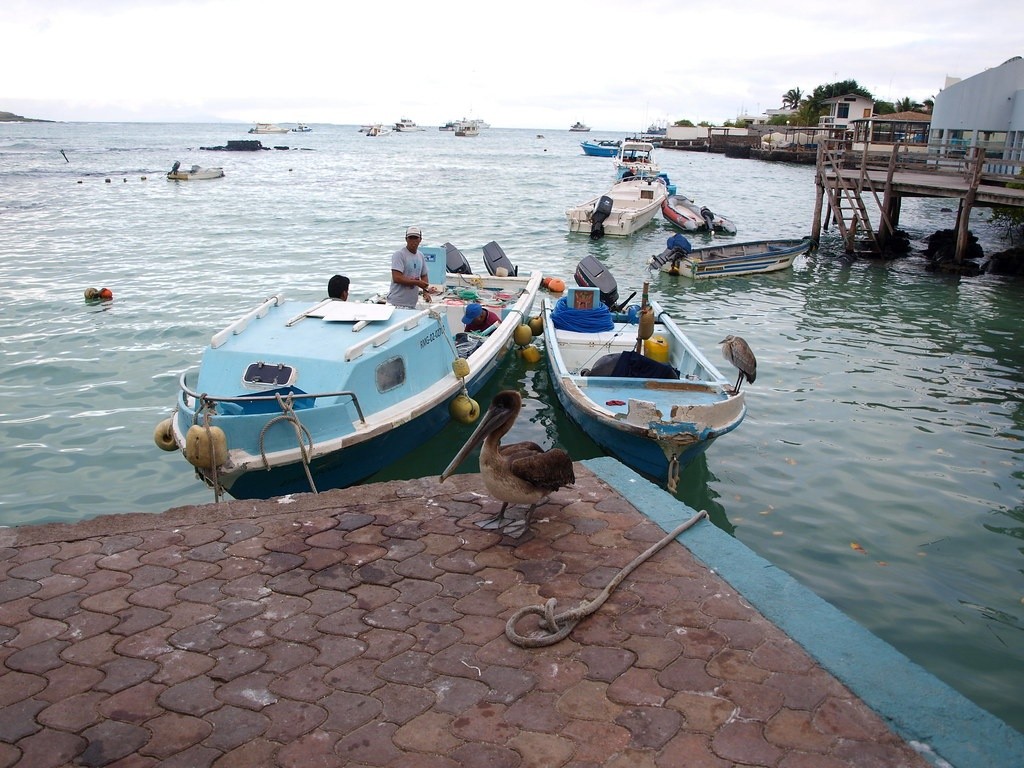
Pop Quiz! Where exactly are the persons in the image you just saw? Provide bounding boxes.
[174,161,180,175]
[462,304,501,336]
[328,275,350,302]
[622,167,636,182]
[386,226,432,310]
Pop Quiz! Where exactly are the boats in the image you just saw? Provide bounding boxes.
[580,135,678,197]
[292,121,312,134]
[153,240,542,497]
[649,231,812,281]
[167,160,226,181]
[249,122,290,134]
[359,118,426,137]
[540,255,745,479]
[435,117,491,137]
[565,171,668,239]
[662,192,739,238]
[568,122,591,133]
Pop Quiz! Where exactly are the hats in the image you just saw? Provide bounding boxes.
[328,275,350,293]
[462,302,481,325]
[406,227,421,239]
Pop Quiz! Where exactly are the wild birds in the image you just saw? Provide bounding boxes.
[717,334,757,396]
[439,391,576,540]
